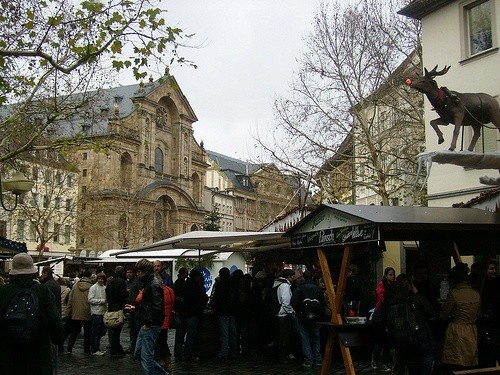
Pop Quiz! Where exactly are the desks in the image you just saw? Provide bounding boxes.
[193,310,220,359]
[315,318,385,362]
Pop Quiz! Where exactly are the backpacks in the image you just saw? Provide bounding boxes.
[297,287,322,326]
[5,281,43,337]
[264,281,284,315]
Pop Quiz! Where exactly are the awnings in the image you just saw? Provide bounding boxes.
[109,230,285,260]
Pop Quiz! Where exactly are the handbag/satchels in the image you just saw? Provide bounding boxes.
[103,309,125,327]
[169,312,182,329]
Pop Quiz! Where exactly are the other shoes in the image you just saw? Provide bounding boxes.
[111,350,125,356]
[316,360,324,366]
[101,350,106,353]
[91,351,104,355]
[380,364,391,372]
[67,351,71,354]
[371,361,377,369]
[303,361,312,367]
[84,350,91,354]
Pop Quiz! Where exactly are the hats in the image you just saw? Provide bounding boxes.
[9,252,39,275]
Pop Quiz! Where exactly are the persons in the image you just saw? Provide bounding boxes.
[0,254,500,375]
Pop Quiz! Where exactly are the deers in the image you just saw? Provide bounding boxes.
[408,65,500,152]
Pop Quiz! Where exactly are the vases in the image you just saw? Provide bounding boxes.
[345,317,367,325]
